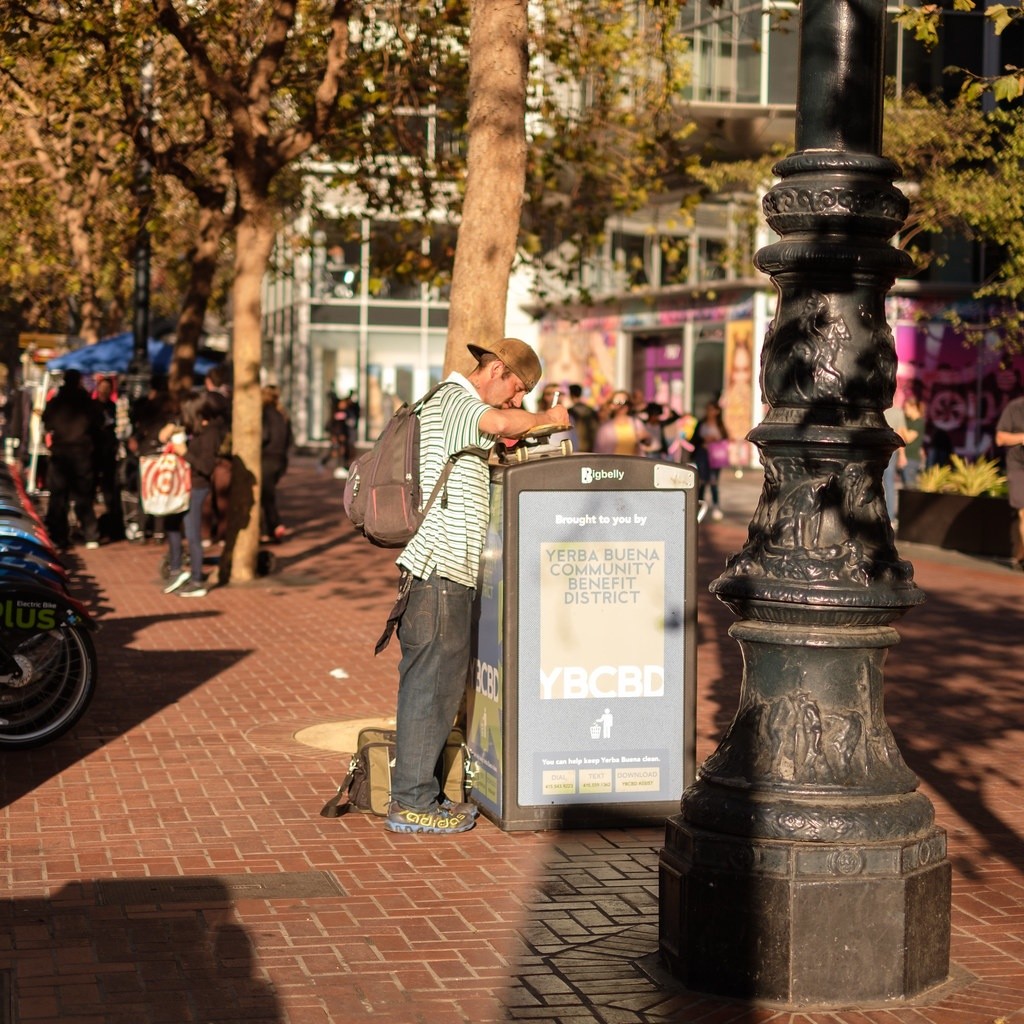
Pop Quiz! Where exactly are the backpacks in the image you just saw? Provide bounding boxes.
[343,382,491,550]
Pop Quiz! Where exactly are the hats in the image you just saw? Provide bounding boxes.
[468,338,542,392]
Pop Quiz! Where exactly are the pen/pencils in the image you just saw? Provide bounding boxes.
[550,391,560,409]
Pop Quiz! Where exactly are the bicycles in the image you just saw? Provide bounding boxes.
[1,459,99,752]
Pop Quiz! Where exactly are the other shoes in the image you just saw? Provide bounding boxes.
[85,541,99,549]
[180,586,208,597]
[712,504,724,521]
[274,524,296,539]
[163,570,192,592]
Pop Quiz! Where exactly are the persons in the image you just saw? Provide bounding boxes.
[371,338,571,835]
[489,377,729,526]
[994,394,1024,569]
[1,362,403,601]
[881,392,1015,532]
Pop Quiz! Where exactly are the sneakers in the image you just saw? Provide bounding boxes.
[384,798,475,834]
[440,799,480,820]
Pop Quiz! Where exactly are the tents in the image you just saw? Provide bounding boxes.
[24,329,233,497]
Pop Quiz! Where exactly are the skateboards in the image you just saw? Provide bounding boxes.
[496,422,574,460]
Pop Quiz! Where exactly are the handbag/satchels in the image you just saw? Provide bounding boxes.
[139,441,191,516]
[705,438,730,467]
[321,726,473,817]
